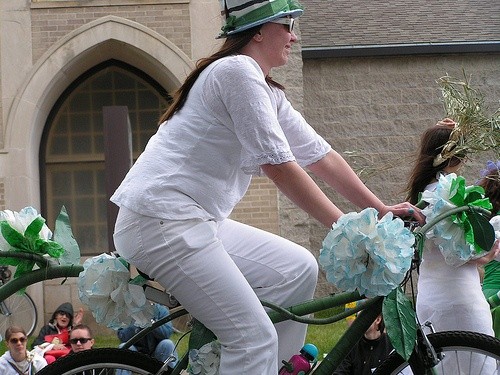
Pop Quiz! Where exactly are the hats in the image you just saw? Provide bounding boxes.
[216,0,303,39]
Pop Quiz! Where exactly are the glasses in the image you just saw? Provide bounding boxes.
[9,337,27,343]
[269,18,295,33]
[69,338,92,344]
[60,312,71,319]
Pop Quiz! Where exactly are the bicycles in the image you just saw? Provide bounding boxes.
[0,264,39,345]
[37,199,500,375]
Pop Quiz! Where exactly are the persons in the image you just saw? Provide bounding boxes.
[65,324,116,375]
[31,301,84,375]
[468,161,500,341]
[109,1,425,375]
[404,118,500,375]
[115,262,180,375]
[0,324,34,375]
[344,292,414,375]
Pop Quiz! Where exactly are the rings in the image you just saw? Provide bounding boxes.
[408,209,414,215]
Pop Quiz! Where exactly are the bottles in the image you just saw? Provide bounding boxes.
[278,343,318,375]
